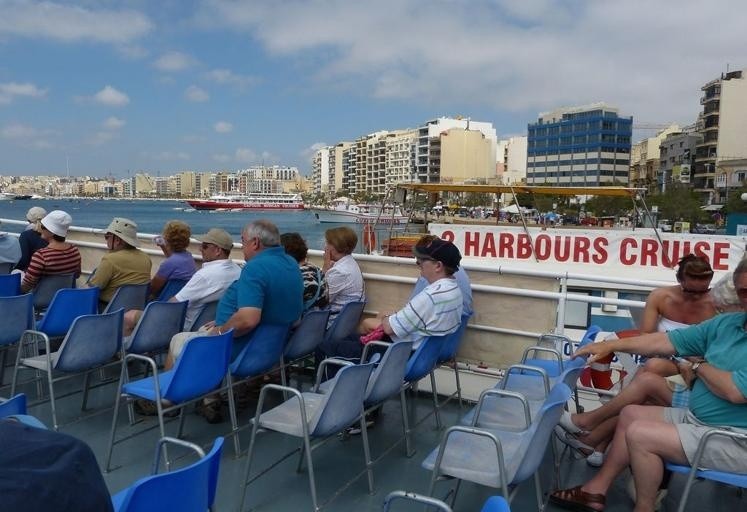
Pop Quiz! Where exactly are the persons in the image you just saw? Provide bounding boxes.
[430,201,645,229]
[133,218,303,422]
[574,255,714,466]
[81,218,152,313]
[13,207,48,270]
[0,417,114,512]
[221,232,326,408]
[148,220,197,305]
[553,270,746,459]
[321,226,365,330]
[11,210,82,293]
[317,240,462,438]
[544,260,747,511]
[409,235,471,328]
[123,228,241,339]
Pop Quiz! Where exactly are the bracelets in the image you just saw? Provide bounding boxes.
[691,357,705,371]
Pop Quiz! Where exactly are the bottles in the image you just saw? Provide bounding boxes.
[412,239,461,267]
[107,218,141,247]
[190,228,233,251]
[40,210,72,237]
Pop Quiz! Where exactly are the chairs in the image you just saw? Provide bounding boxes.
[0,273,475,512]
[380,326,604,511]
[195,399,223,423]
[134,399,180,417]
[666,429,746,511]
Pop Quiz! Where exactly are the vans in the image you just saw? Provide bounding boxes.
[553,411,605,466]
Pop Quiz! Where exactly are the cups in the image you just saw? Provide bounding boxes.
[681,284,711,293]
[105,233,116,239]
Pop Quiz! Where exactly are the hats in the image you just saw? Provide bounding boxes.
[434,196,675,231]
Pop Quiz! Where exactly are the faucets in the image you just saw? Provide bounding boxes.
[545,486,606,512]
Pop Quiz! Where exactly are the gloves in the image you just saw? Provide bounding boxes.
[309,203,406,226]
[359,180,678,274]
[187,189,304,213]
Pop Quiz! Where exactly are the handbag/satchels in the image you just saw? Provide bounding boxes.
[691,357,705,371]
